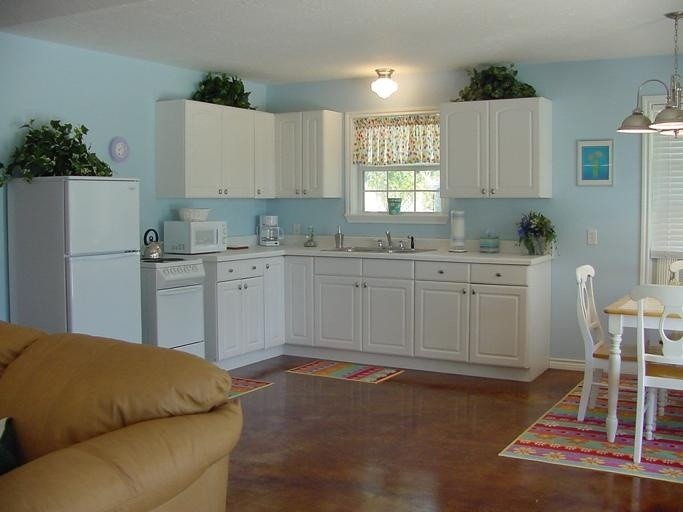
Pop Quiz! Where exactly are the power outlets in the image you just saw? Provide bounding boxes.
[587,231,598,245]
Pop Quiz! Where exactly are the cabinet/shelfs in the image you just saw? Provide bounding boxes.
[414,248,553,384]
[155,99,254,198]
[439,97,553,201]
[313,251,415,356]
[205,251,265,372]
[265,253,286,364]
[255,110,278,198]
[277,109,344,198]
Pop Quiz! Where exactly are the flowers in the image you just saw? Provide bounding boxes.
[512,208,559,255]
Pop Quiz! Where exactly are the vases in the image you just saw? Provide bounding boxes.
[387,197,402,214]
[524,233,551,254]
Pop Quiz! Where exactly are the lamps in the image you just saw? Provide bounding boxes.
[370,68,399,98]
[619,8,683,137]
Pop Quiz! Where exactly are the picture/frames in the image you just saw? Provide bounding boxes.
[578,140,614,185]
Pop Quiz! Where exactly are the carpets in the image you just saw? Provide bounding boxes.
[499,373,683,483]
[230,376,275,398]
[285,360,406,386]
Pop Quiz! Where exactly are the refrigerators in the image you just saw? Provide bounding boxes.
[6,176,142,345]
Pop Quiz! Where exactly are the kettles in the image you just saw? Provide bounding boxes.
[141,229,164,259]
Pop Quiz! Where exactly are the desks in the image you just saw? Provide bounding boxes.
[602,292,683,443]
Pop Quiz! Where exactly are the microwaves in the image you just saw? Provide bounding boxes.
[162,220,226,254]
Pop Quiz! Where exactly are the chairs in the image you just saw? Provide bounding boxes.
[575,264,661,431]
[628,284,683,464]
[661,258,683,342]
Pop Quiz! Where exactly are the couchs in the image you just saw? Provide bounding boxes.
[0,319,243,512]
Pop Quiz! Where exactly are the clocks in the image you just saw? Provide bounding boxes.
[109,136,130,162]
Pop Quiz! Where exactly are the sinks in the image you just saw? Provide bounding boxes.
[347,245,436,254]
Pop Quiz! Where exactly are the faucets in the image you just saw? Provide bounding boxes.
[385,229,394,248]
[408,234,415,250]
